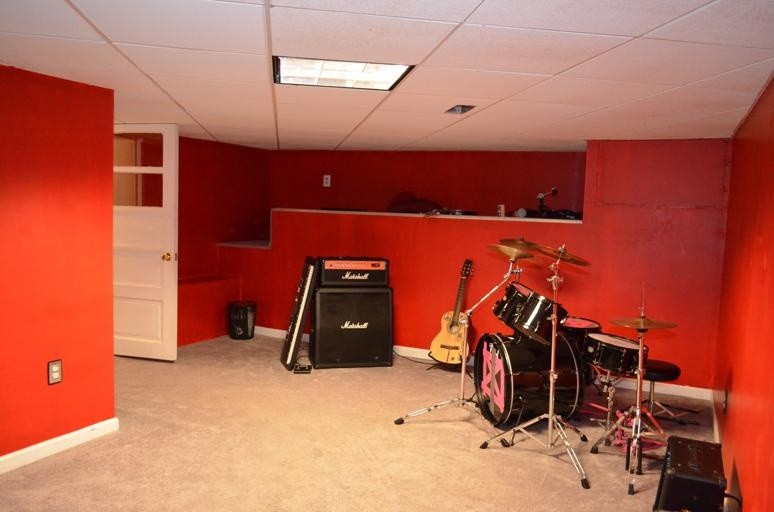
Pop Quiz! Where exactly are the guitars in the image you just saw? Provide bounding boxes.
[431,259,475,366]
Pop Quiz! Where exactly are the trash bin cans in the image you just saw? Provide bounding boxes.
[229,300,256,340]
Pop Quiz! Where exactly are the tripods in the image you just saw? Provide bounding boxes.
[394,261,513,447]
[480,259,591,489]
[590,333,668,495]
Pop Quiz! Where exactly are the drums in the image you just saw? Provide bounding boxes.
[494,281,535,328]
[584,332,648,374]
[474,330,583,427]
[514,292,568,345]
[559,316,600,359]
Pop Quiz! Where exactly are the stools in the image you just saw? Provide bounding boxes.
[640,360,684,427]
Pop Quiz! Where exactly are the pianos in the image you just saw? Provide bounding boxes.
[280,256,317,371]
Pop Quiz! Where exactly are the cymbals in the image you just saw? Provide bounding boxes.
[607,319,677,329]
[500,239,539,249]
[489,244,534,258]
[532,245,591,266]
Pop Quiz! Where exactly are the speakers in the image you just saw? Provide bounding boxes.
[653,435,727,512]
[308,288,393,369]
